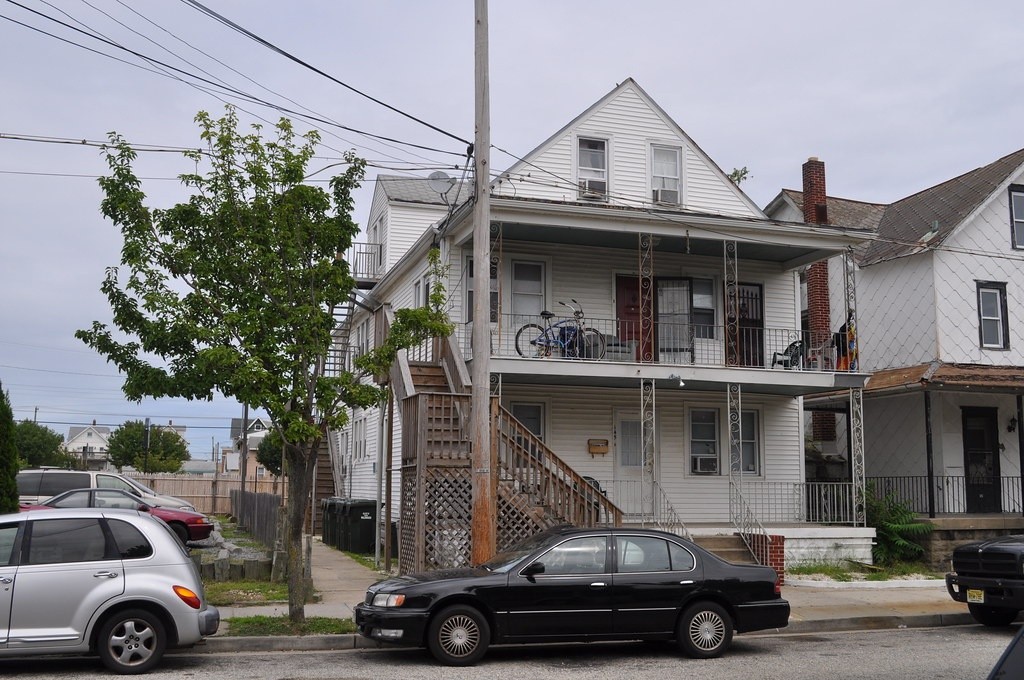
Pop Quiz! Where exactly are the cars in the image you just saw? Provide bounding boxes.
[354,523,791,667]
[945,533,1024,627]
[0,507,221,675]
[18,487,215,547]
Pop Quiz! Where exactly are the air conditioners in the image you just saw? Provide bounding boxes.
[655,189,680,205]
[582,179,607,198]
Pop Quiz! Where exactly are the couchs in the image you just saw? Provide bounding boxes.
[586,334,638,362]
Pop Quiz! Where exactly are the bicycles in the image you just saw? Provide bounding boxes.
[515,299,608,362]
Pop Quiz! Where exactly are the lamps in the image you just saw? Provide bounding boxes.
[1007,414,1017,433]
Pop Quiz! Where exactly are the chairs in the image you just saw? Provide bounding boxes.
[771,340,805,370]
[809,338,837,371]
[574,476,609,523]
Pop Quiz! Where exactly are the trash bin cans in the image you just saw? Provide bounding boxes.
[334,499,356,551]
[560,326,583,359]
[321,497,350,546]
[347,499,385,553]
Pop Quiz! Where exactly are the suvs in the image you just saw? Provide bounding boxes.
[16,467,198,515]
